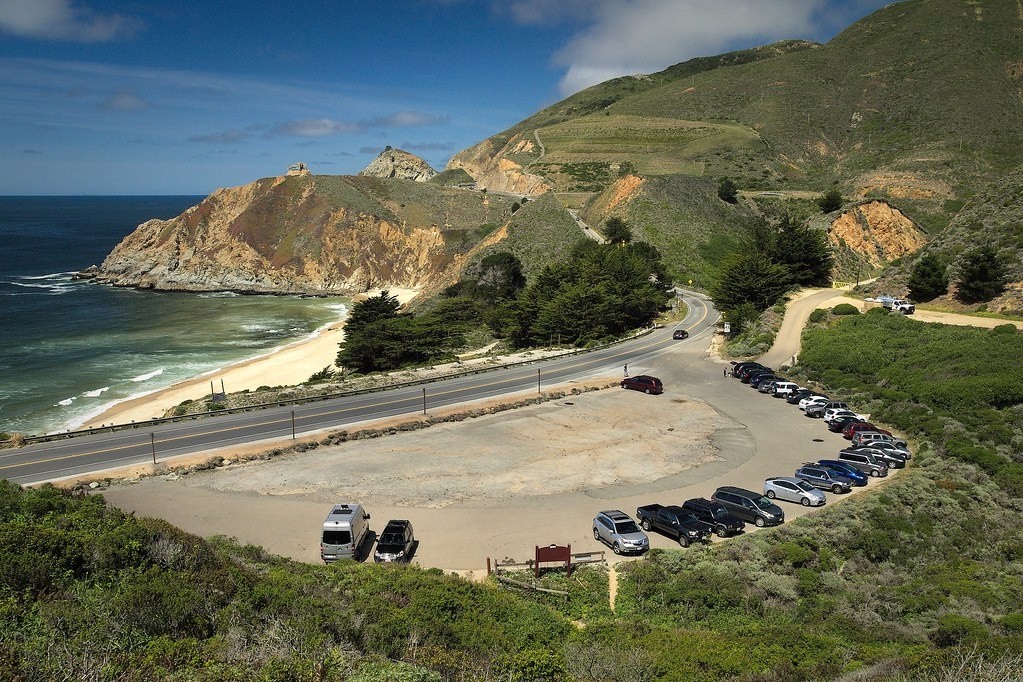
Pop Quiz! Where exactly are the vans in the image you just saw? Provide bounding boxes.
[320,504,370,564]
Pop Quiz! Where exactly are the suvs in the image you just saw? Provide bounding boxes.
[593,510,649,556]
[794,465,852,494]
[619,374,663,394]
[682,497,746,538]
[730,361,809,399]
[806,401,849,418]
[711,486,784,528]
[838,449,889,477]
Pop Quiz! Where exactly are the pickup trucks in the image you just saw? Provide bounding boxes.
[882,298,915,314]
[636,504,712,548]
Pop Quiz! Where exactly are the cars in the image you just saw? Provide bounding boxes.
[848,431,911,468]
[673,330,688,340]
[824,408,892,440]
[373,519,414,566]
[763,477,826,507]
[819,460,868,486]
[787,389,829,411]
